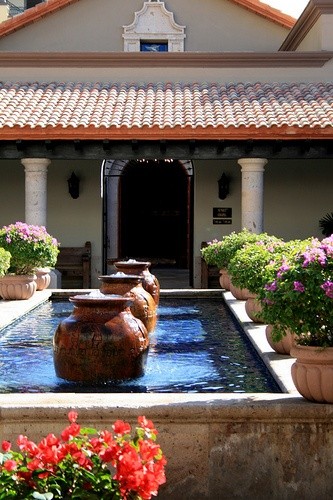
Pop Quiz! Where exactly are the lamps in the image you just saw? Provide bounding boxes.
[217,173,232,200]
[66,172,80,199]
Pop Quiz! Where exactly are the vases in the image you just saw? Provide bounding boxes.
[245,297,265,323]
[230,282,258,300]
[0,274,37,300]
[218,269,232,291]
[291,337,333,404]
[265,321,310,355]
[30,268,51,291]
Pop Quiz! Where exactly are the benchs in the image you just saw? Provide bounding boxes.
[55,241,91,289]
[201,241,223,288]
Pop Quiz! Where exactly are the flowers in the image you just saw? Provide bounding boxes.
[199,227,333,347]
[0,221,59,276]
